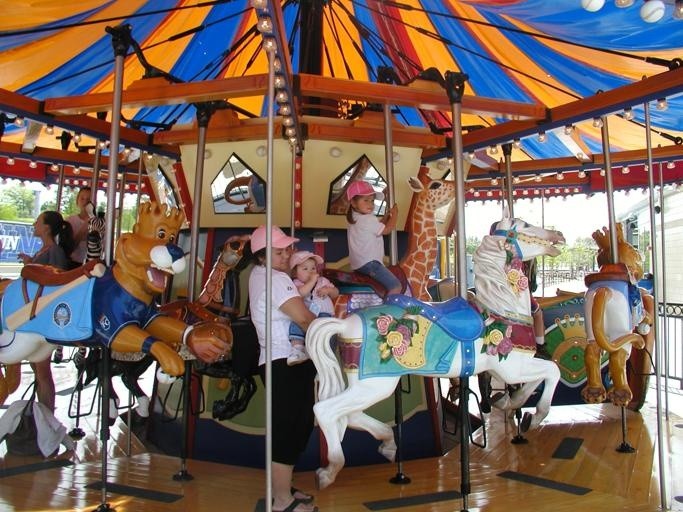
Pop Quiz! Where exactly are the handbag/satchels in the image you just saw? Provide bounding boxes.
[4,415,41,456]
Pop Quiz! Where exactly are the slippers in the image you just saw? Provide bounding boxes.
[272,486,319,512]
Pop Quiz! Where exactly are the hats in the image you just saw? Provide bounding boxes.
[250,223,301,254]
[347,181,385,201]
[289,250,324,270]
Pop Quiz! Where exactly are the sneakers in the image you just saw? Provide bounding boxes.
[286,344,309,366]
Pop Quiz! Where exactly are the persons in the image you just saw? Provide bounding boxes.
[287,250,339,366]
[490,222,552,360]
[248,225,319,512]
[347,181,403,296]
[64,186,93,271]
[18,210,73,415]
